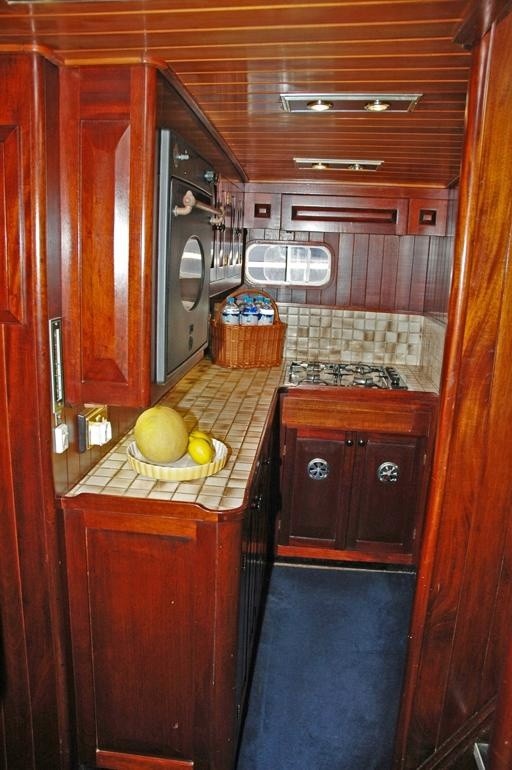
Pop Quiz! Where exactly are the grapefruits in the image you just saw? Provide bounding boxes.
[134,406,188,462]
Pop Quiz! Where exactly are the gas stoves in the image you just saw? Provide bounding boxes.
[282,360,408,389]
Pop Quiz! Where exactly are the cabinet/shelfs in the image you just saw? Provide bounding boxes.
[243,184,458,235]
[61,420,278,768]
[211,178,243,295]
[275,388,429,564]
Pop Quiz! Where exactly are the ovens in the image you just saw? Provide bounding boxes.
[155,131,223,385]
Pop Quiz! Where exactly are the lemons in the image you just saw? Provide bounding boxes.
[189,431,216,457]
[188,436,212,463]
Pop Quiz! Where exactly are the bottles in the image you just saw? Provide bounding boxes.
[221,294,275,326]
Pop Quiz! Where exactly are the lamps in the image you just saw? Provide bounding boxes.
[281,94,422,114]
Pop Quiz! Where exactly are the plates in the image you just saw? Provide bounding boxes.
[126,438,228,481]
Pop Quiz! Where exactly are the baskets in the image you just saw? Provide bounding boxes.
[211,289,288,370]
